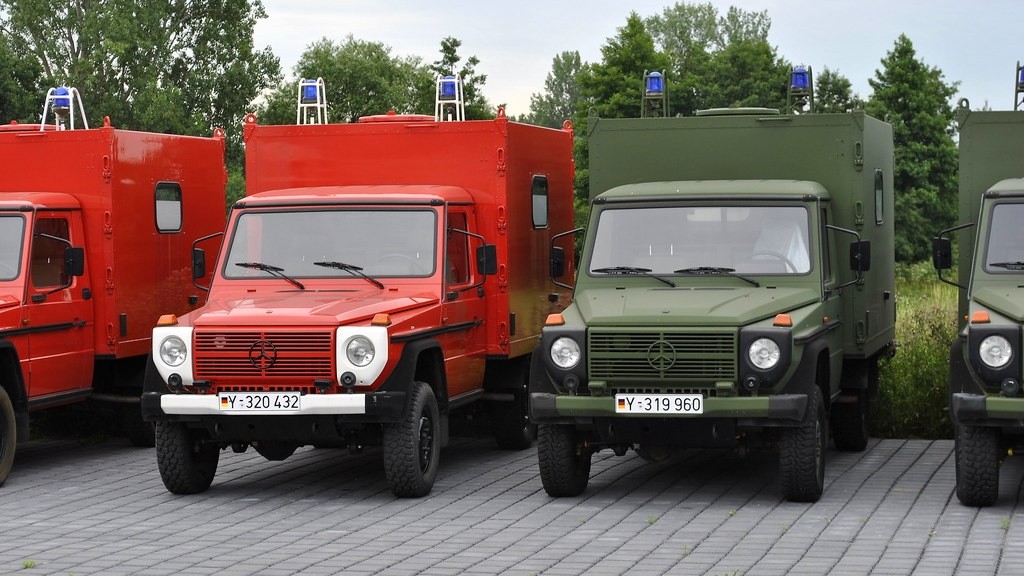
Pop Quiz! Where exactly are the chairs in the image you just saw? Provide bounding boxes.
[635,213,690,274]
[752,220,811,273]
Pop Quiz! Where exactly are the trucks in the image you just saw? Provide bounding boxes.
[0,86,229,487]
[141,75,577,497]
[933,62,1024,506]
[527,66,898,504]
